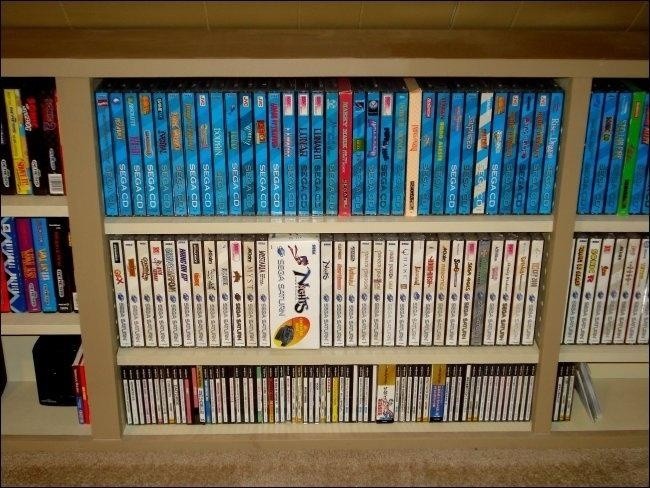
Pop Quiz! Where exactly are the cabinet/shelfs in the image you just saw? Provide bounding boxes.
[0,58,93,452]
[89,59,572,450]
[550,60,650,449]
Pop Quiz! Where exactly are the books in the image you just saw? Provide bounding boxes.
[561,231,650,345]
[96,82,564,218]
[577,83,650,216]
[122,364,537,424]
[553,361,602,422]
[1,77,88,427]
[110,234,545,347]
